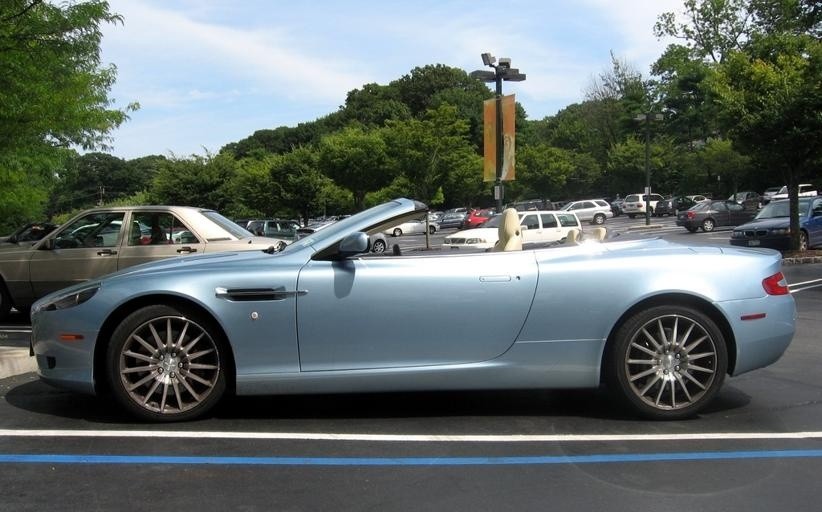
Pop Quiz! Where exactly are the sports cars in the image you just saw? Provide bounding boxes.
[27,196,798,423]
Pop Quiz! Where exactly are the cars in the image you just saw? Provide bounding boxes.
[596,182,819,231]
[246,205,494,252]
[556,200,612,226]
[730,198,822,252]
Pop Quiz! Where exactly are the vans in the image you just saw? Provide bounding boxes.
[443,211,582,250]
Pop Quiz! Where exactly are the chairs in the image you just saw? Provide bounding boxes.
[489,204,607,254]
[128,222,168,246]
